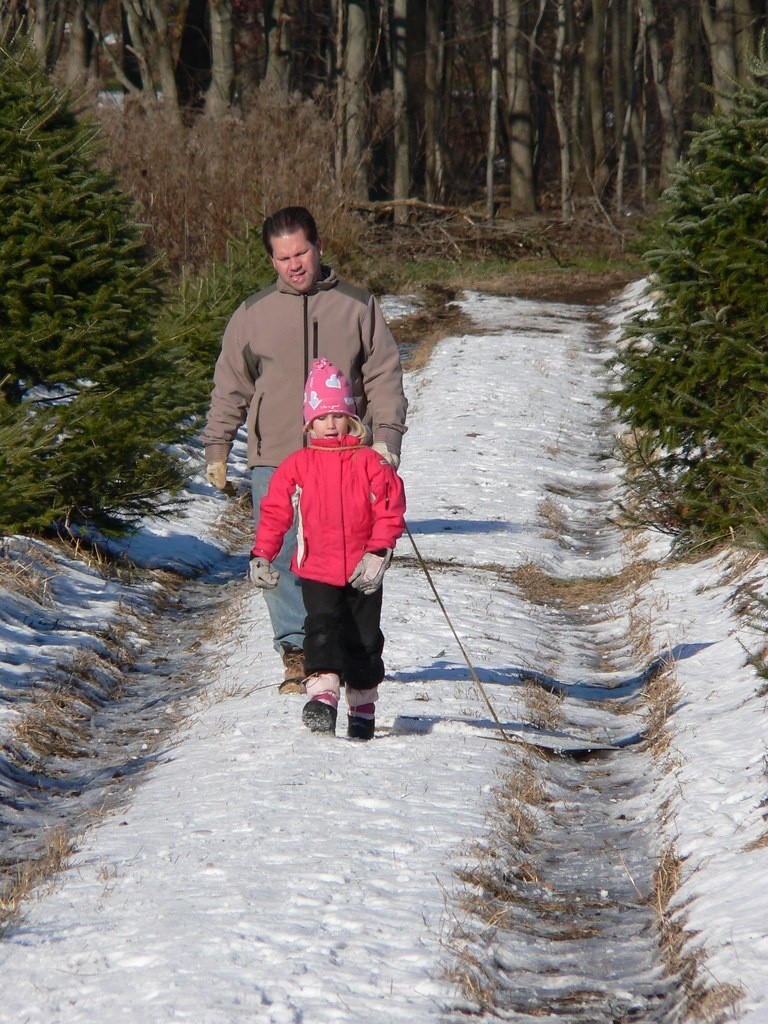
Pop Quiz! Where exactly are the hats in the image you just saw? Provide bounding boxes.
[301,358,359,433]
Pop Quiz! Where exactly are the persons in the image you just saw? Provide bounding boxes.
[249,357,406,741]
[202,207,408,695]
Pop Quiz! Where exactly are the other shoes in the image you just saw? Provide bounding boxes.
[348,706,375,740]
[278,651,307,694]
[302,698,338,735]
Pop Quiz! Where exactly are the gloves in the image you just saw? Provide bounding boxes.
[349,548,394,594]
[383,450,400,470]
[207,462,228,488]
[249,557,280,589]
